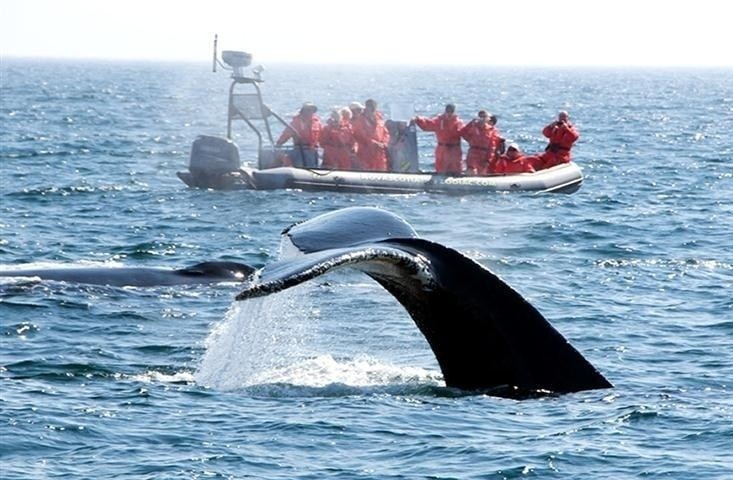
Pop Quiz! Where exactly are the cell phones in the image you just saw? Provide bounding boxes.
[556,122,563,125]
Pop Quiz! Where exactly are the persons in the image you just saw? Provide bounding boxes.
[413,102,465,174]
[490,143,538,176]
[487,115,500,149]
[458,111,496,175]
[276,95,392,173]
[541,111,578,166]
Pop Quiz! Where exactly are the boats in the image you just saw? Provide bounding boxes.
[176,161,584,198]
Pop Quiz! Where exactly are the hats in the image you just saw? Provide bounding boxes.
[350,102,366,110]
[506,143,520,154]
[300,101,317,114]
